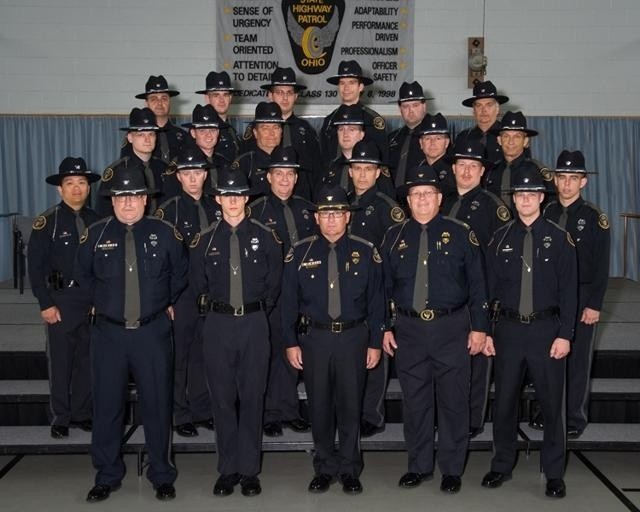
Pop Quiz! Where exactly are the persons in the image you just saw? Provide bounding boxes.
[434,140,515,438]
[190,169,283,496]
[387,81,433,186]
[240,66,318,157]
[318,60,389,149]
[317,103,392,203]
[94,108,173,219]
[486,112,557,217]
[279,188,387,494]
[72,172,187,501]
[28,156,106,440]
[121,75,189,166]
[529,149,611,438]
[454,80,510,167]
[401,114,462,215]
[232,102,311,203]
[153,147,222,437]
[381,167,493,493]
[249,146,323,436]
[339,140,408,436]
[194,70,240,158]
[159,104,235,202]
[481,161,578,496]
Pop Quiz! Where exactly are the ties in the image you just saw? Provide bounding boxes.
[142,161,157,216]
[229,228,243,310]
[124,225,142,325]
[159,127,170,165]
[281,199,300,246]
[227,125,237,143]
[519,227,533,318]
[206,156,218,190]
[194,199,208,232]
[395,129,414,189]
[501,162,512,212]
[558,208,569,231]
[283,123,292,149]
[346,195,362,235]
[75,212,86,237]
[327,242,341,321]
[340,158,351,194]
[479,131,487,152]
[412,225,429,314]
[448,195,464,220]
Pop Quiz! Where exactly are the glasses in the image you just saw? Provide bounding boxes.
[255,125,282,133]
[272,89,296,97]
[317,210,348,218]
[114,195,143,203]
[197,128,218,135]
[408,188,441,198]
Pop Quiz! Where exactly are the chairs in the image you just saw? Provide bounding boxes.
[11,214,38,292]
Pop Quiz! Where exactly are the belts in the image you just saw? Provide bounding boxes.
[207,299,264,316]
[48,279,79,288]
[394,301,468,322]
[498,305,559,326]
[305,314,367,334]
[94,308,166,328]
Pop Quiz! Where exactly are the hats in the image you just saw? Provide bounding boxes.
[110,68,308,197]
[326,60,374,86]
[462,80,509,107]
[46,156,102,185]
[310,106,546,209]
[548,150,597,174]
[388,80,435,103]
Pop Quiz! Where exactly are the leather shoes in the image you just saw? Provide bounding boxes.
[51,425,69,439]
[399,471,434,488]
[529,411,544,431]
[69,418,94,432]
[153,481,176,501]
[440,474,461,493]
[309,474,339,493]
[469,427,483,440]
[176,415,385,439]
[545,478,566,497]
[567,419,587,440]
[337,473,362,495]
[86,480,121,502]
[213,472,242,496]
[239,474,262,497]
[481,470,512,488]
